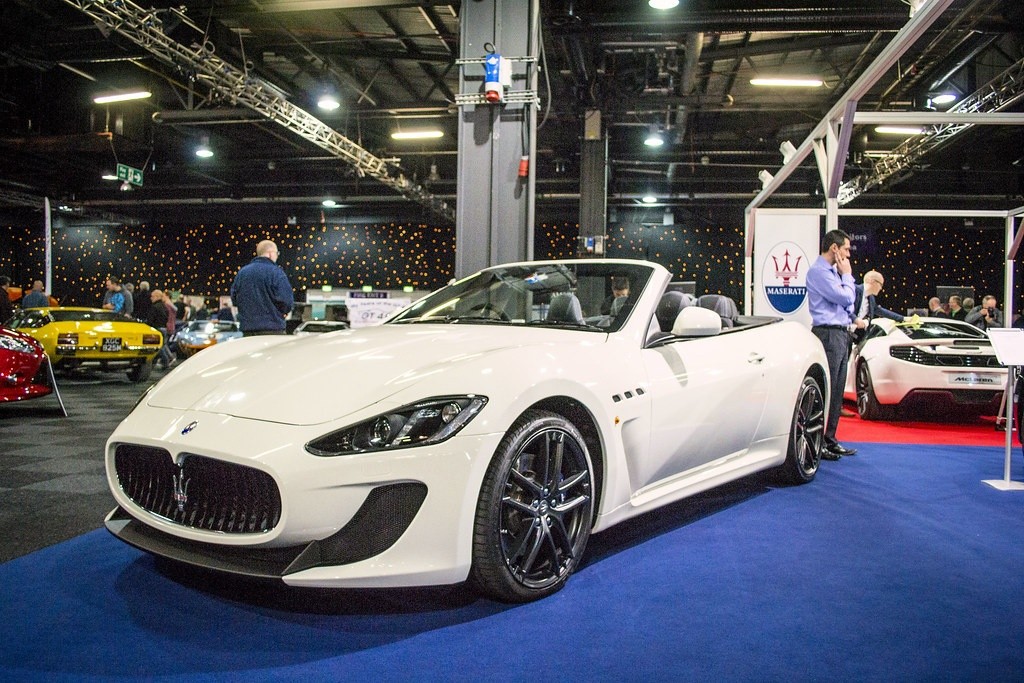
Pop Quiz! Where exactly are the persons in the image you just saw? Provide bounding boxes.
[805,230,857,460]
[231,240,293,336]
[601,277,630,315]
[103,277,233,372]
[22,281,48,308]
[929,295,1024,332]
[840,271,911,417]
[0,276,13,323]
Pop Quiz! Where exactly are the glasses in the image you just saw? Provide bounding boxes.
[875,280,884,290]
[271,251,281,256]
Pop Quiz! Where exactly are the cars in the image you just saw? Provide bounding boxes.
[103,257,842,606]
[174,319,245,357]
[0,323,54,403]
[293,320,349,336]
[843,317,1022,422]
[2,306,164,383]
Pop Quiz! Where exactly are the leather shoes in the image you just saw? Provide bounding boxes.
[821,448,840,461]
[828,444,857,455]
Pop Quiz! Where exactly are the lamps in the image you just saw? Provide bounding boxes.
[778,140,797,164]
[644,124,664,145]
[196,144,214,157]
[392,118,444,139]
[758,170,773,189]
[318,94,340,108]
[749,74,823,86]
[93,86,152,103]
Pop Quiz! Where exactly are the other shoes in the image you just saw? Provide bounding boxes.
[169,356,176,365]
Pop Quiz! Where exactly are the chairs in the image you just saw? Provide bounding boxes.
[697,294,734,328]
[598,296,627,326]
[547,293,585,326]
[647,292,692,346]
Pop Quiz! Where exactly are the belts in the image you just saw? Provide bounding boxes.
[818,324,851,333]
